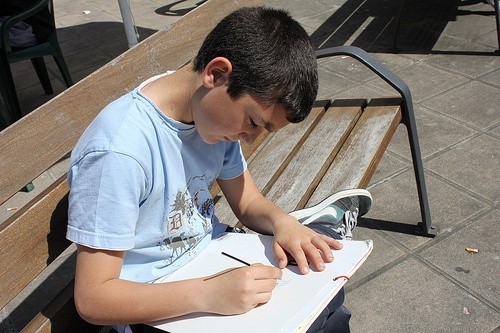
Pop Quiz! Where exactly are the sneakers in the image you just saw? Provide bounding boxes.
[285,187,374,234]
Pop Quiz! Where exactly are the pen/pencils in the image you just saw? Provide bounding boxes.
[221,251,251,266]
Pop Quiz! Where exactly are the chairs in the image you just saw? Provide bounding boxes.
[0,0,75,192]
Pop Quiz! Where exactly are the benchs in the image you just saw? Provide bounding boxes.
[1,0,437,332]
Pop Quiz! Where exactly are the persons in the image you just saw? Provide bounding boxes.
[68,5,374,332]
